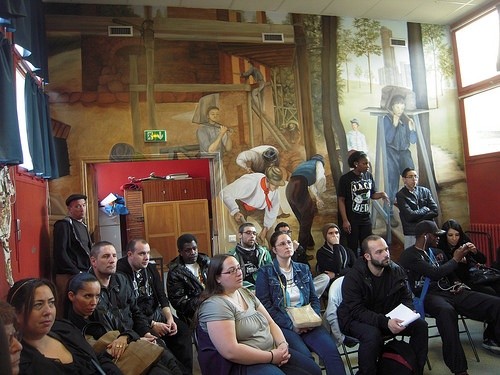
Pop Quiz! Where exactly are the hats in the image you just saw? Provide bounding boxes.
[414,220,446,235]
[323,223,340,241]
[66,194,87,206]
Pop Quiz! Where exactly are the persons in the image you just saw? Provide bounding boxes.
[116,236,193,375]
[166,233,211,351]
[192,253,323,375]
[87,241,186,375]
[0,299,23,375]
[337,151,391,258]
[228,223,272,294]
[53,195,93,305]
[396,167,439,251]
[7,278,124,375]
[397,220,500,375]
[337,235,429,375]
[255,231,347,375]
[316,223,357,293]
[268,222,311,273]
[63,271,141,375]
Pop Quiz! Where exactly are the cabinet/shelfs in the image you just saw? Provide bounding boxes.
[124,178,211,272]
[0,164,48,297]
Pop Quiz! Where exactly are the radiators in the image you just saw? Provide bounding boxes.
[469,223,500,261]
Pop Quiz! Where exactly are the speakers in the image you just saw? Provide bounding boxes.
[53,136,70,177]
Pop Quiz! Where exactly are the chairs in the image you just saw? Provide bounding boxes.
[193,297,486,375]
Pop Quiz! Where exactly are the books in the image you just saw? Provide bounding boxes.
[286,305,322,327]
[166,173,189,179]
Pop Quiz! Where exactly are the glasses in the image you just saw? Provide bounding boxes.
[220,266,241,275]
[282,230,292,234]
[241,231,258,235]
[404,175,419,178]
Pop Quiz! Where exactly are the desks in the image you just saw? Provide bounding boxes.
[150,247,164,281]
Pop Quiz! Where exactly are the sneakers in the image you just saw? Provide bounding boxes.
[482,339,500,350]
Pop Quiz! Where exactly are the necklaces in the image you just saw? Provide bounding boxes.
[230,292,242,307]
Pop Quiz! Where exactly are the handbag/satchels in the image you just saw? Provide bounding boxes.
[82,321,164,375]
[470,266,500,285]
[287,304,322,328]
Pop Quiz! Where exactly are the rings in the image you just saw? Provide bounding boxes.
[115,344,120,348]
[120,345,123,348]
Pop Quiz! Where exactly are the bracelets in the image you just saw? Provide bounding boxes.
[151,321,155,328]
[269,350,273,363]
[278,340,288,346]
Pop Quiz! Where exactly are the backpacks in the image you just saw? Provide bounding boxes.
[376,340,431,375]
